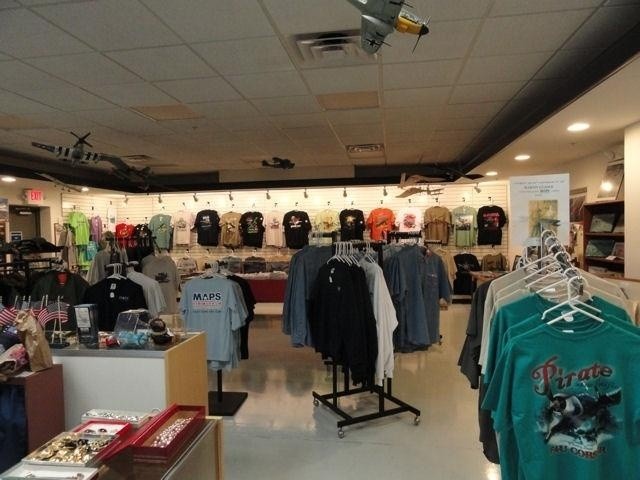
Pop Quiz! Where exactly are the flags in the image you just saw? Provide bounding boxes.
[0,300,68,327]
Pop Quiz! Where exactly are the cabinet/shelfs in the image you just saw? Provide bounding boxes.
[48,330,209,434]
[0,363,65,458]
[583,200,624,281]
[89,414,224,480]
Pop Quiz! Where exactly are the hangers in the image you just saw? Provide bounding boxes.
[297,229,449,275]
[185,261,244,286]
[40,234,167,287]
[515,233,636,348]
